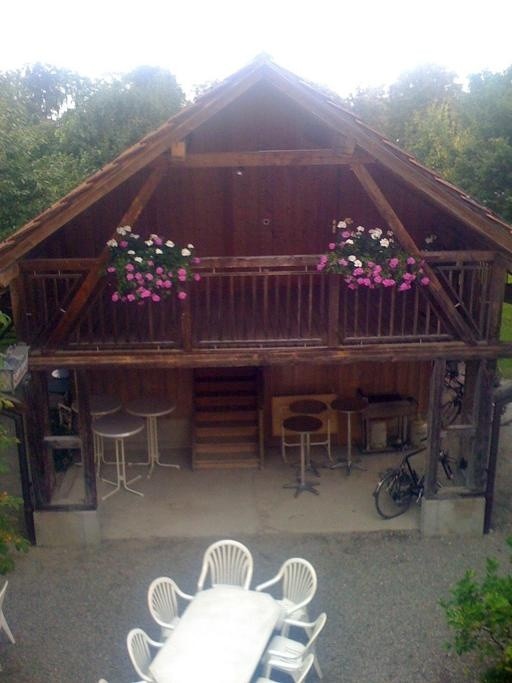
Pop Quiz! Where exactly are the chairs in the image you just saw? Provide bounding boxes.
[0,580,16,645]
[126,626,163,682]
[255,555,319,643]
[146,575,195,632]
[265,611,327,682]
[255,650,316,682]
[197,538,254,591]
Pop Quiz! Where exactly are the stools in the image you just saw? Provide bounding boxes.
[287,397,329,478]
[328,395,369,477]
[283,412,323,499]
[71,396,127,479]
[90,412,147,501]
[123,396,181,480]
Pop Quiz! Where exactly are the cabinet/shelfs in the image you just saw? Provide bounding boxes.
[357,392,420,456]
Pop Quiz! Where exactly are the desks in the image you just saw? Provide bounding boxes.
[146,583,282,683]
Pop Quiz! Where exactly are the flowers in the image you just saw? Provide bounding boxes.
[102,222,205,309]
[312,212,431,297]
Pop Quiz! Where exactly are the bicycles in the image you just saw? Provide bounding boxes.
[441,368,512,432]
[372,429,489,520]
